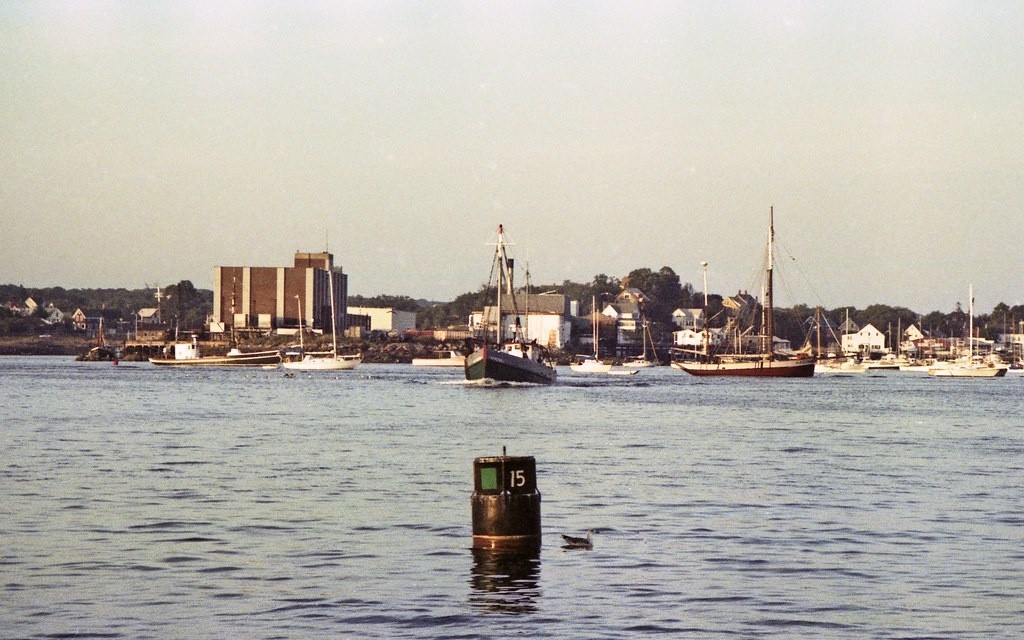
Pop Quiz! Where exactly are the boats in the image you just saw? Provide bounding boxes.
[280,351,365,369]
[463,223,558,385]
[411,351,465,367]
[609,369,640,376]
[148,349,282,372]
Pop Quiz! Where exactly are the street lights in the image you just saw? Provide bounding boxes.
[702,259,710,358]
[293,294,305,349]
[130,312,139,343]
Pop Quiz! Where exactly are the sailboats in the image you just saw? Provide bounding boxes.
[570,293,596,365]
[622,314,665,368]
[569,290,612,374]
[817,287,1024,379]
[677,207,817,378]
